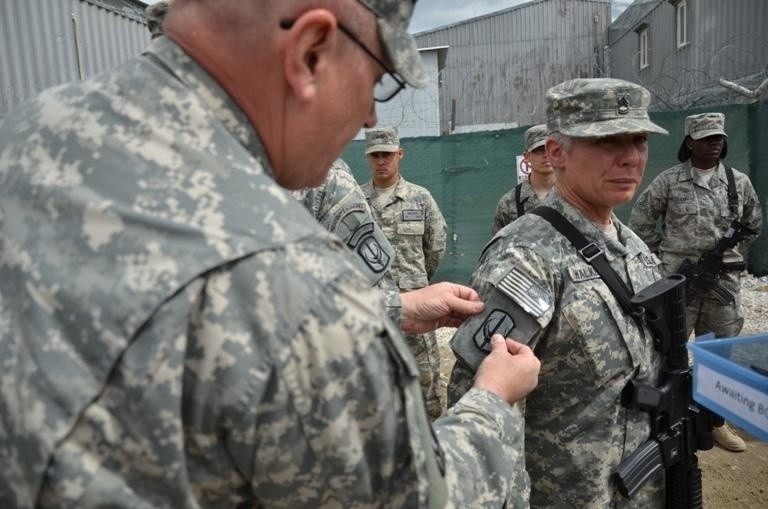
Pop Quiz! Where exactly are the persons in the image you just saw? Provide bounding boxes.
[281,155,400,324]
[0,0,543,509]
[353,123,448,425]
[448,74,708,509]
[489,120,556,236]
[624,110,766,455]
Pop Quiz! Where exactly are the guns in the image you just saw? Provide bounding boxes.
[676,197,757,302]
[611,269,724,509]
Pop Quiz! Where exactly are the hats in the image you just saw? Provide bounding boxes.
[363,125,400,155]
[543,77,670,140]
[523,123,548,158]
[351,0,430,90]
[684,112,730,141]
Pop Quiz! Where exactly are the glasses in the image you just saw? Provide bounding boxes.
[276,13,408,103]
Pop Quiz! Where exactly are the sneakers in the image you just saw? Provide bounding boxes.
[713,417,747,453]
